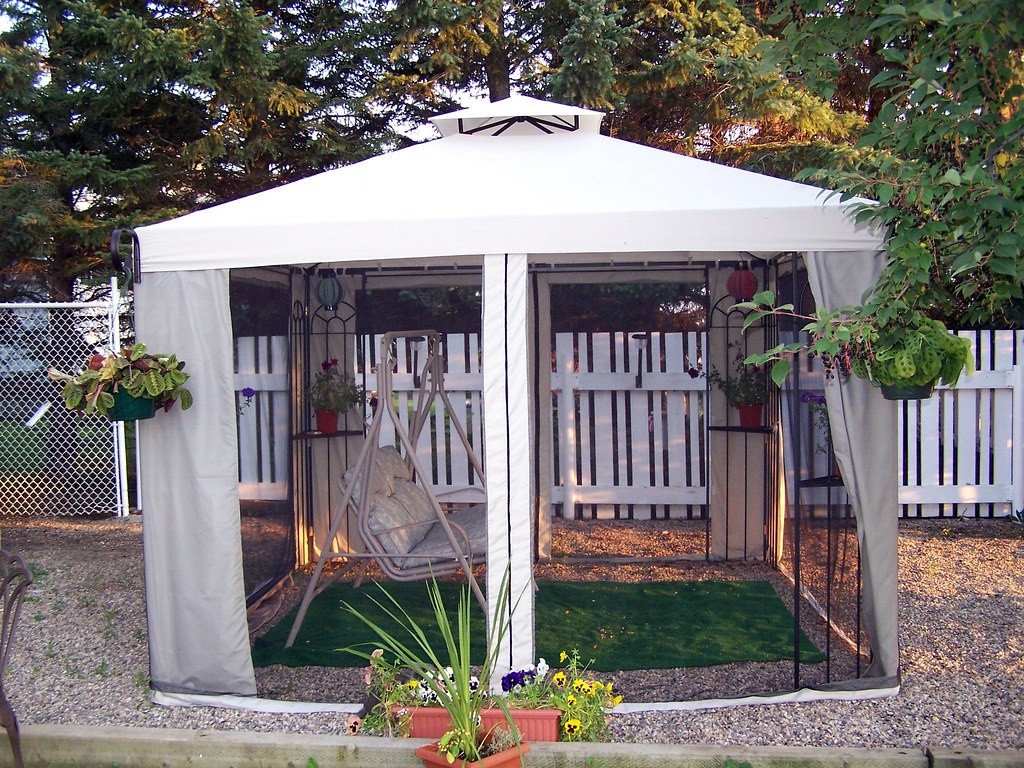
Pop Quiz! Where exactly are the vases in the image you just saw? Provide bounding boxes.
[392,705,562,741]
[832,455,842,477]
[736,404,762,427]
[314,408,338,433]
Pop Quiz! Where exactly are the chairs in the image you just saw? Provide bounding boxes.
[0,549,34,768]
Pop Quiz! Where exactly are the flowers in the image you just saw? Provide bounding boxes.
[349,649,623,760]
[309,358,371,418]
[688,341,769,408]
[236,388,255,416]
[801,392,834,456]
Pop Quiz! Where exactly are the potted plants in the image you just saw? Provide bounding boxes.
[334,556,532,768]
[47,344,194,421]
[813,303,972,400]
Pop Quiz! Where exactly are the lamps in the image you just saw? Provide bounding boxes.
[313,268,344,311]
